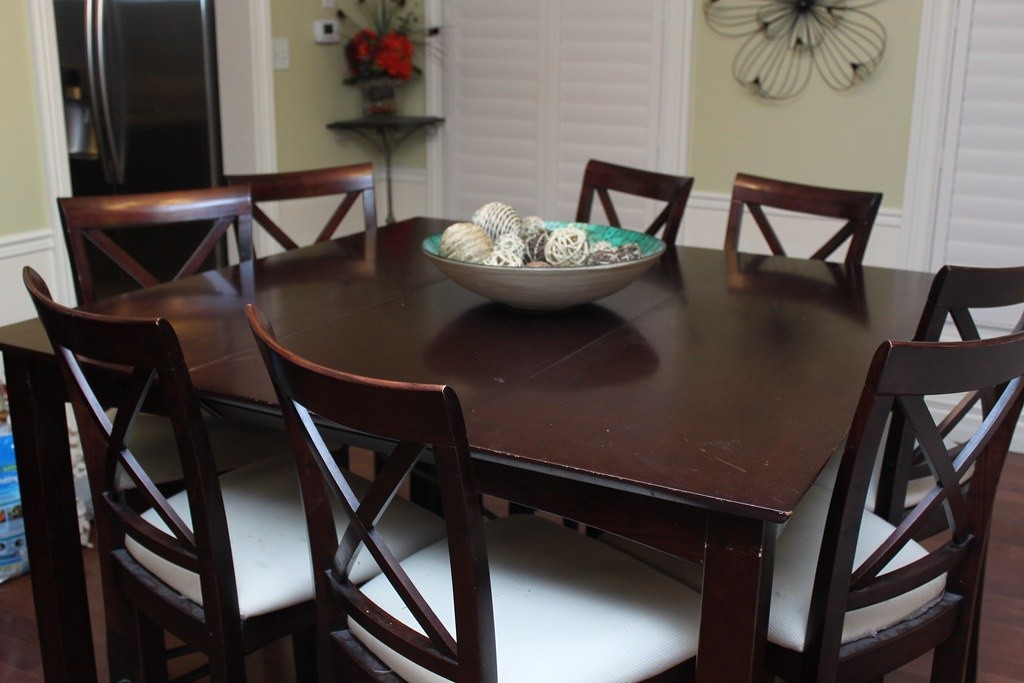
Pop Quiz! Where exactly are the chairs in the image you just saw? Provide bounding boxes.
[246,301,702,682]
[766,330,1024,683]
[54,185,350,513]
[814,263,1024,542]
[221,162,444,518]
[723,173,884,266]
[510,160,695,540]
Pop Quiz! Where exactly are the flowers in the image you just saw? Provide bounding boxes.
[337,1,442,85]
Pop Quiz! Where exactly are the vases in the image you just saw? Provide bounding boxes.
[355,78,404,121]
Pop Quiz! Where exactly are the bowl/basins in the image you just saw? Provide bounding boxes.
[421,222,666,308]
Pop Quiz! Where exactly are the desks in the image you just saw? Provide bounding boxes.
[0,217,937,683]
